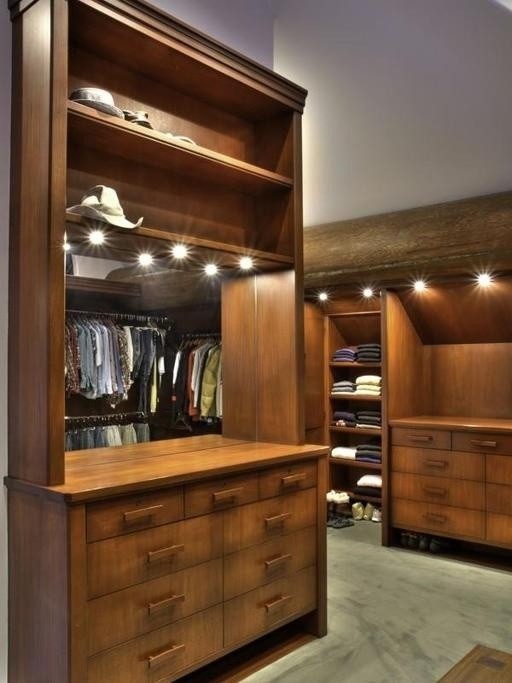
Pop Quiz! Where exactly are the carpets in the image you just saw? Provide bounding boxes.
[437,637,512,682]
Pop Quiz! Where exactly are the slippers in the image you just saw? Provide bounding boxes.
[326,516,354,528]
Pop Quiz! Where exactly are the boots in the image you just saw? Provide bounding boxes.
[326,489,352,517]
[352,502,375,521]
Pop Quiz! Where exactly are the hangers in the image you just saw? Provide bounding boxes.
[176,329,222,348]
[62,305,169,335]
[57,410,152,433]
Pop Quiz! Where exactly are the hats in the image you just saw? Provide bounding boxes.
[123,109,153,131]
[66,184,143,231]
[68,87,126,121]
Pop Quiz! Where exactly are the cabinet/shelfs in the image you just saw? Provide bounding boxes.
[385,412,511,553]
[322,304,388,526]
[3,428,331,682]
[1,0,315,487]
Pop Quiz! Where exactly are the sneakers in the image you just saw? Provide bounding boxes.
[371,508,382,522]
